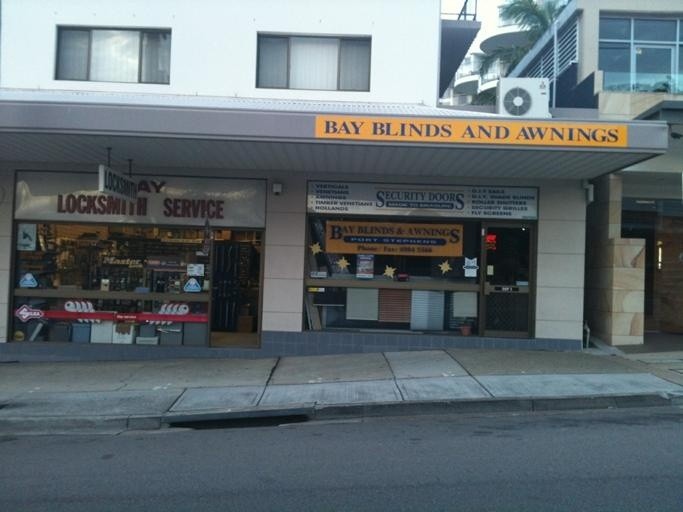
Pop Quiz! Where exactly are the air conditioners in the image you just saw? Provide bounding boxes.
[496,77,550,119]
